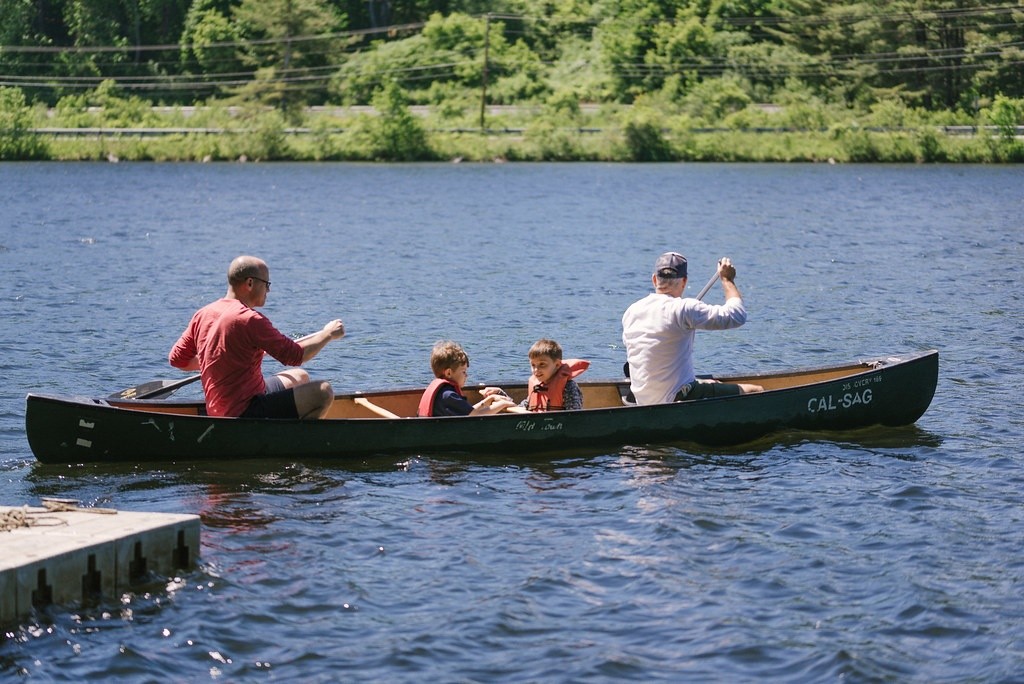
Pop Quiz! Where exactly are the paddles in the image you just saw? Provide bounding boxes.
[623,272,720,376]
[113,330,325,400]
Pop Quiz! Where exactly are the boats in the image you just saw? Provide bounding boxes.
[24,349,939,464]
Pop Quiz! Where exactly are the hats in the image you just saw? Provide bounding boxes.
[653,252,690,279]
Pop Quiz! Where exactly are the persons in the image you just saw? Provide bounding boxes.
[169,256,344,419]
[416,339,516,417]
[482,338,583,411]
[622,251,763,405]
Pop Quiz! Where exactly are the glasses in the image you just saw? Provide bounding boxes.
[251,276,271,288]
